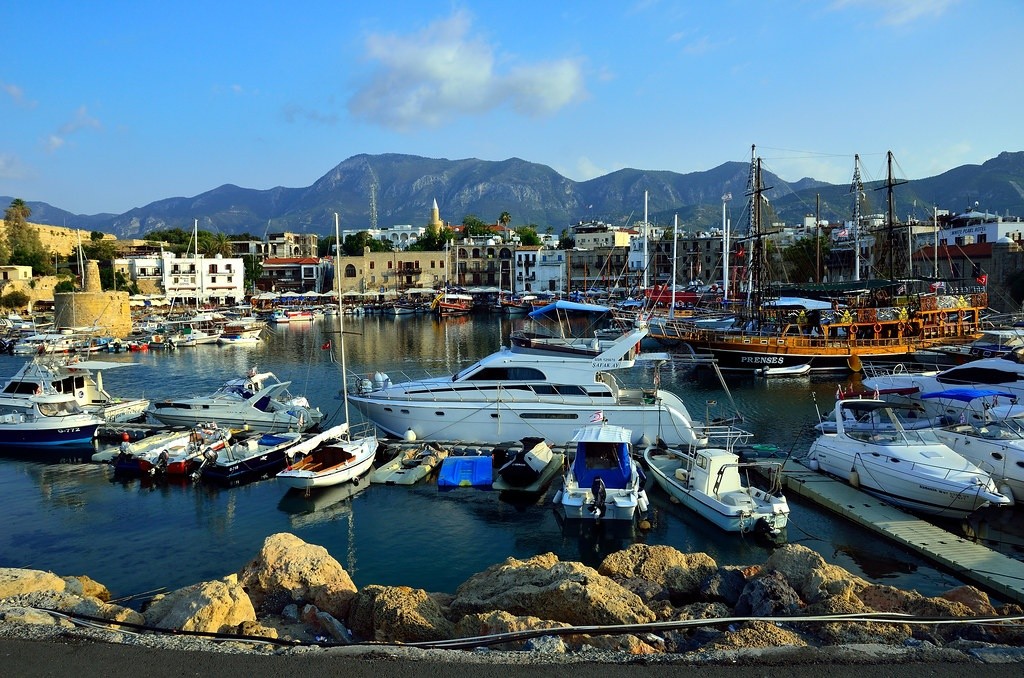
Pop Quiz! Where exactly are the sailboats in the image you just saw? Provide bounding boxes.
[275,213,379,491]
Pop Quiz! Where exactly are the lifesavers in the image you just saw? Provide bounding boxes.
[957,309,966,318]
[849,324,858,334]
[897,322,905,331]
[873,323,882,333]
[716,297,721,302]
[678,300,682,306]
[906,324,913,332]
[667,303,671,309]
[939,311,948,321]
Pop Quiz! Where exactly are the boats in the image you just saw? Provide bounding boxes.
[552,388,651,526]
[0,140,1024,512]
[369,444,448,485]
[140,364,323,434]
[644,362,789,540]
[91,422,233,478]
[0,285,151,450]
[327,209,745,451]
[188,413,305,484]
[438,455,493,487]
[805,384,1011,520]
[490,436,564,496]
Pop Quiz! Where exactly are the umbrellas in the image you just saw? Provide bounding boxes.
[28,301,32,313]
[470,286,644,296]
[250,290,403,308]
[130,289,241,305]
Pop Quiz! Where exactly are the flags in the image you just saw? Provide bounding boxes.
[736,248,744,257]
[321,342,330,350]
[590,411,604,422]
[898,287,903,295]
[977,276,987,286]
[932,282,944,289]
[837,229,848,237]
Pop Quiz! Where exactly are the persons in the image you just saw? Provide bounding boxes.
[39,307,45,312]
